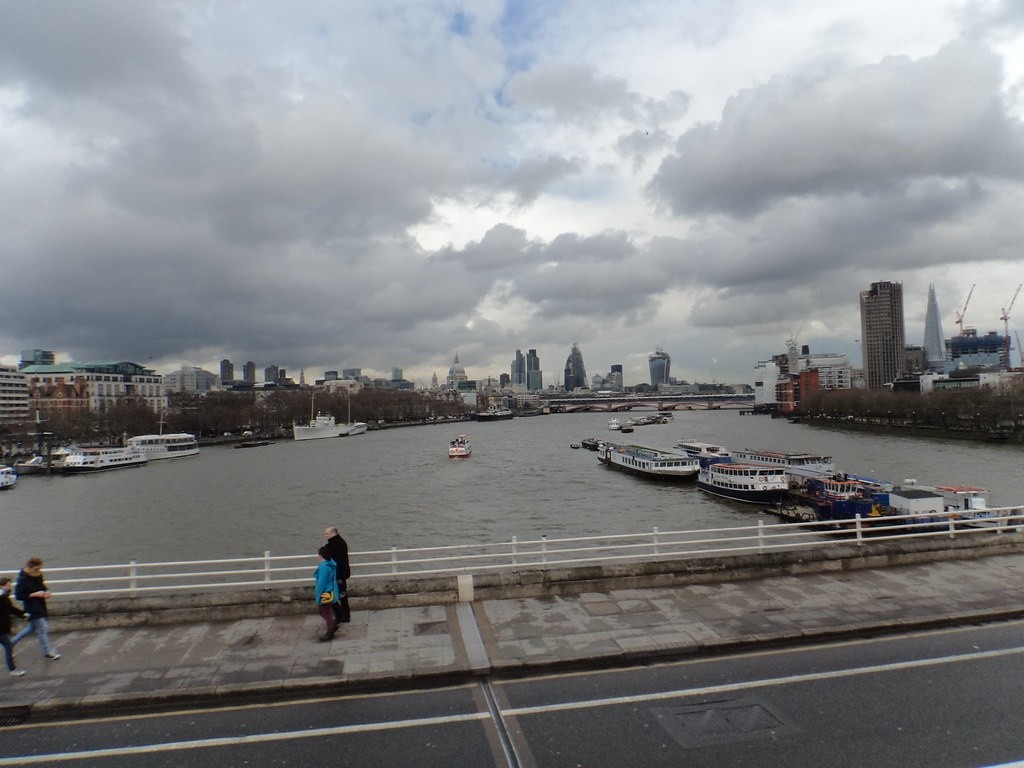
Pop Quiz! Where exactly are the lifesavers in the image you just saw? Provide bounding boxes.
[780,476,786,482]
[763,486,767,491]
[759,477,764,482]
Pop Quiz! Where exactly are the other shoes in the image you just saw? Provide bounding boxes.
[335,615,342,624]
[43,654,61,660]
[333,623,339,632]
[320,632,335,641]
[340,617,350,622]
[8,669,25,676]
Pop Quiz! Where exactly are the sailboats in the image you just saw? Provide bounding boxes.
[293,389,369,442]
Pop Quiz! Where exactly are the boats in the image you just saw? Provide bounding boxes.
[125,415,201,461]
[596,440,702,481]
[674,437,734,469]
[697,447,996,538]
[581,436,601,452]
[608,409,675,434]
[470,403,515,422]
[447,435,472,458]
[11,409,149,475]
[570,442,580,449]
[232,439,274,449]
[0,465,18,489]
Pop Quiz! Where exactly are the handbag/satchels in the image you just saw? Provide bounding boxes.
[320,590,334,604]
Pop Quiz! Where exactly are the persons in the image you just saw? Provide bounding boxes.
[325,526,351,624]
[10,557,60,661]
[0,577,30,677]
[313,547,340,642]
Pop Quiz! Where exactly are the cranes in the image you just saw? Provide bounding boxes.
[953,284,979,339]
[998,282,1023,373]
